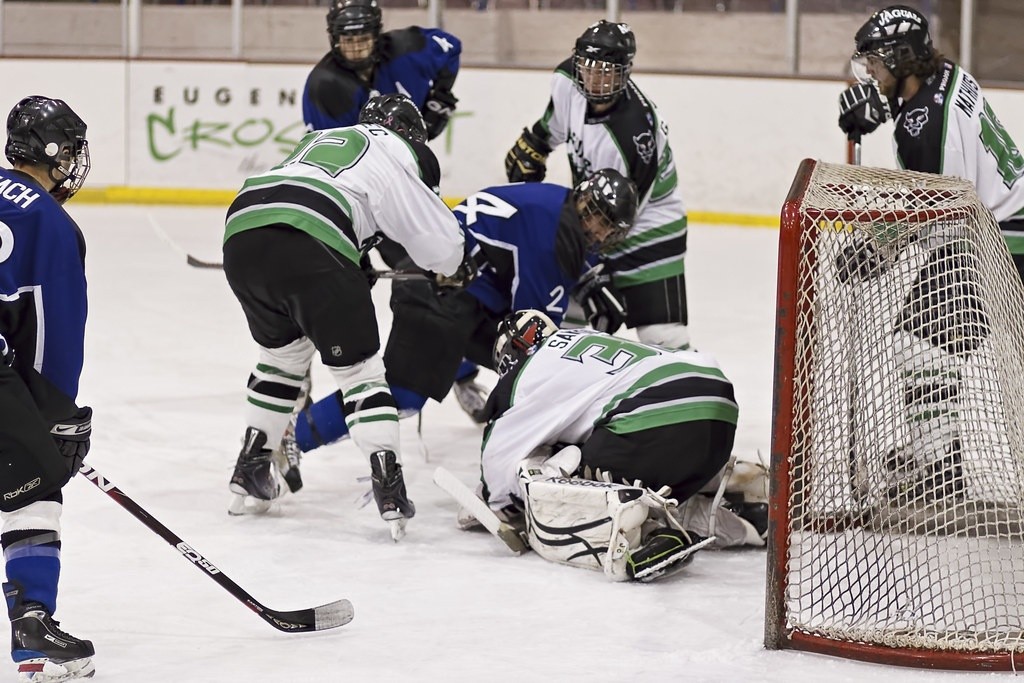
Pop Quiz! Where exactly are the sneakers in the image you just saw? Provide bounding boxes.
[628,528,699,580]
[727,502,768,536]
[272,420,302,493]
[882,436,968,505]
[370,450,416,541]
[226,427,282,514]
[2,581,96,681]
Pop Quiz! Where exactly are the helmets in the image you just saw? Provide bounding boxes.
[492,310,560,379]
[850,4,932,79]
[5,96,90,202]
[357,93,428,145]
[576,20,636,103]
[325,0,382,71]
[567,168,636,256]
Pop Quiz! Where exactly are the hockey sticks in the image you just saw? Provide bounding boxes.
[810,275,865,534]
[80,460,356,633]
[433,467,531,557]
[152,225,466,283]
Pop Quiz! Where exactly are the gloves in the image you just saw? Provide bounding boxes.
[50,406,90,476]
[361,253,378,289]
[573,273,626,335]
[423,254,475,298]
[504,127,552,182]
[837,84,887,142]
[423,91,459,141]
[837,238,888,283]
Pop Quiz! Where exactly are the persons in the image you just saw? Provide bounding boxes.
[506,22,689,354]
[221,93,466,544]
[272,168,640,496]
[459,308,768,581]
[1,95,100,682]
[301,0,462,274]
[834,4,1024,502]
